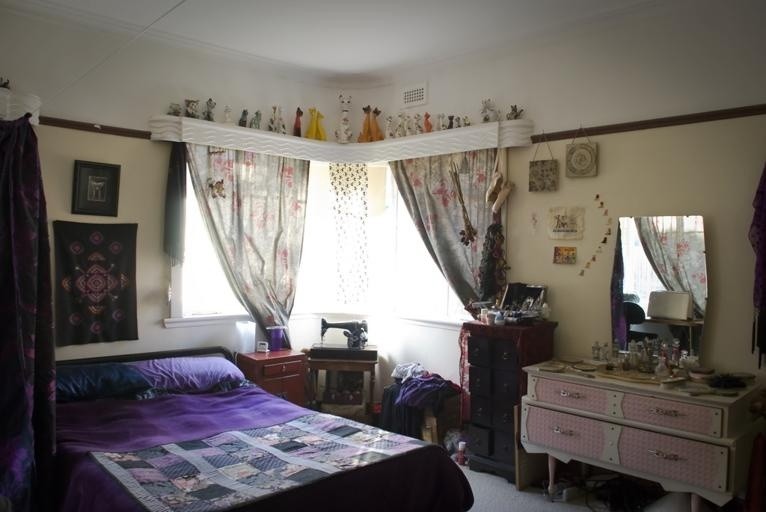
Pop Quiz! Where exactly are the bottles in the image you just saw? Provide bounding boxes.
[592,338,695,378]
[360,330,368,349]
[492,306,508,320]
[458,441,466,466]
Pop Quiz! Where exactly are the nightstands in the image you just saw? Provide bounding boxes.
[240,348,304,406]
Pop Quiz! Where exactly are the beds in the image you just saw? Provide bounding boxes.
[55,347,474,511]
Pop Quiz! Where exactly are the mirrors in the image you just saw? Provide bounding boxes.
[620,213,708,369]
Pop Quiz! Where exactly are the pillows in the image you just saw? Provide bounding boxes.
[56,356,246,401]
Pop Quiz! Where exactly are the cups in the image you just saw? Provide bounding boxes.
[271,329,282,351]
[481,309,496,325]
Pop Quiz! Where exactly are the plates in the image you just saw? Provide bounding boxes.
[574,364,596,371]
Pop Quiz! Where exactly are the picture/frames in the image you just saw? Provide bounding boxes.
[70,161,121,219]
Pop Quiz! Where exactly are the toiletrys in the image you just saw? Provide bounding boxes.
[591,335,689,378]
[480,308,506,325]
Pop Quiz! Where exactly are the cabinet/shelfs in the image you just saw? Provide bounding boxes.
[463,319,557,483]
[519,357,759,512]
